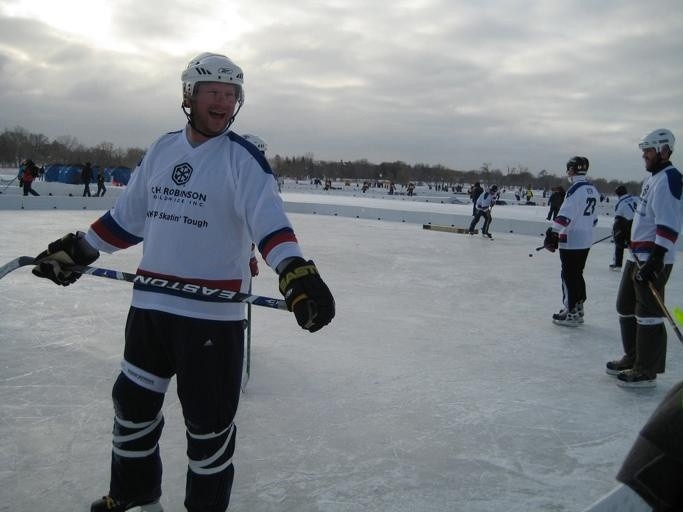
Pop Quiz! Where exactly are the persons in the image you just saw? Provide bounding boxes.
[607,185,638,267]
[309,179,612,222]
[467,183,497,238]
[16,158,44,196]
[31,50,337,512]
[81,161,93,197]
[584,380,682,511]
[604,129,683,384]
[544,155,600,321]
[96,168,106,197]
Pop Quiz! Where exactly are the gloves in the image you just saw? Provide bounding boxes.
[637,250,664,284]
[614,219,633,247]
[484,211,490,218]
[639,129,675,156]
[32,232,99,286]
[279,257,335,332]
[490,201,495,207]
[544,232,559,253]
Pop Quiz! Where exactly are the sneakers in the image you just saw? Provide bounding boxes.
[606,356,634,371]
[89,491,163,512]
[483,232,492,236]
[560,304,584,318]
[469,232,474,236]
[617,368,657,382]
[552,308,578,320]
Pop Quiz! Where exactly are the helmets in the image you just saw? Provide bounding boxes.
[181,53,245,109]
[615,184,628,199]
[489,185,498,195]
[565,157,588,182]
[240,134,267,156]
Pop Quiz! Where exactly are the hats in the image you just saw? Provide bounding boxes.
[474,182,480,187]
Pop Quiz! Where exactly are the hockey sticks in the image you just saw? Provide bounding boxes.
[2,256,291,312]
[473,206,492,218]
[528,247,545,259]
